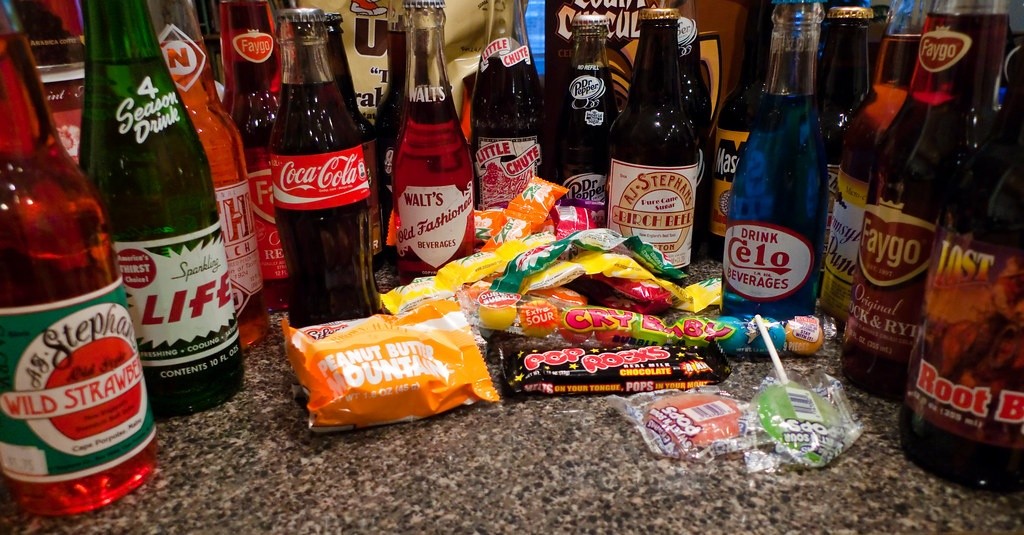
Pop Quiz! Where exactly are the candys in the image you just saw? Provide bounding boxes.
[281,176,864,469]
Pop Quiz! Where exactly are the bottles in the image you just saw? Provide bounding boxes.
[0,0,1024,516]
